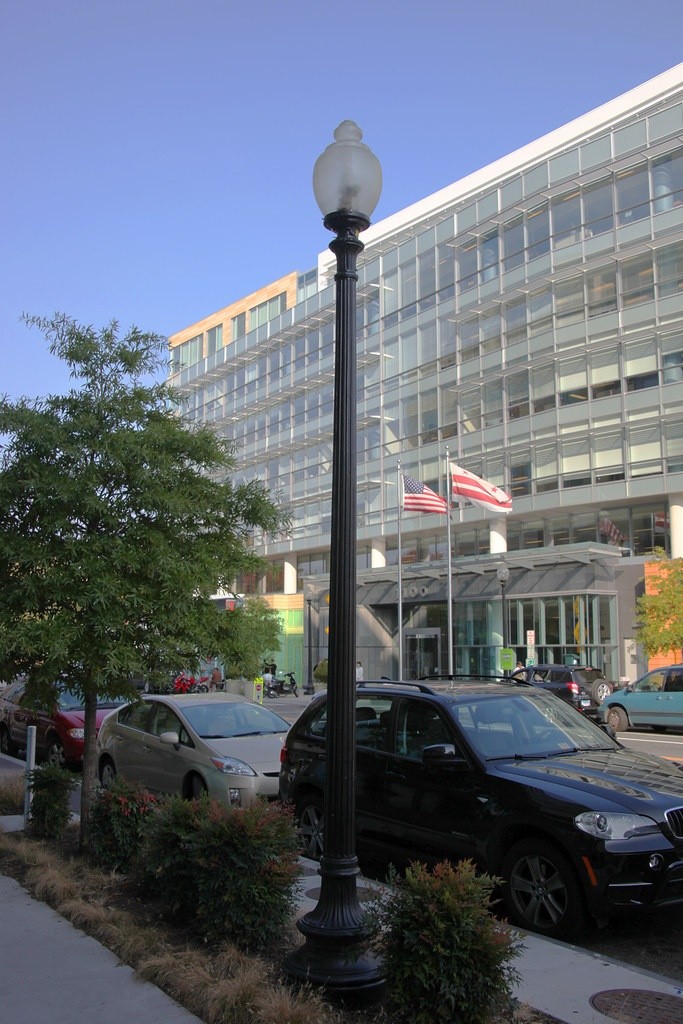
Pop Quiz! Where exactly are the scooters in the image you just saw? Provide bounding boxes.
[263,671,299,698]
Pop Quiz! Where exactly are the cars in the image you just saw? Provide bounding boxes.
[96,692,293,810]
[596,662,683,732]
[0,676,128,771]
[128,669,178,692]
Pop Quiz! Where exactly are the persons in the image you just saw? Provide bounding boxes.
[513,661,524,682]
[262,660,277,677]
[356,662,363,682]
[313,660,321,671]
[210,668,221,692]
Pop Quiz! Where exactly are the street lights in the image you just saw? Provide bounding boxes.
[277,119,402,1010]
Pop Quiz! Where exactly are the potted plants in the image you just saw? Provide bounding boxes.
[225,666,241,693]
[312,661,327,692]
[244,671,258,699]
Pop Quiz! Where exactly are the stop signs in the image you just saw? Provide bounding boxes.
[256,685,262,691]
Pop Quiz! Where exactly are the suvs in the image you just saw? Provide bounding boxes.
[500,665,614,724]
[278,674,683,939]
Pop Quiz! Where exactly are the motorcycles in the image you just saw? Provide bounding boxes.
[171,671,209,694]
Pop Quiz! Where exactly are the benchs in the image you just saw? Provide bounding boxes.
[356,706,393,750]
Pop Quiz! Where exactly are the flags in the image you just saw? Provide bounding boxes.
[603,518,624,542]
[655,516,670,533]
[451,463,512,512]
[402,474,452,519]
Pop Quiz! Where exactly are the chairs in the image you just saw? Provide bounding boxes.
[157,712,186,742]
